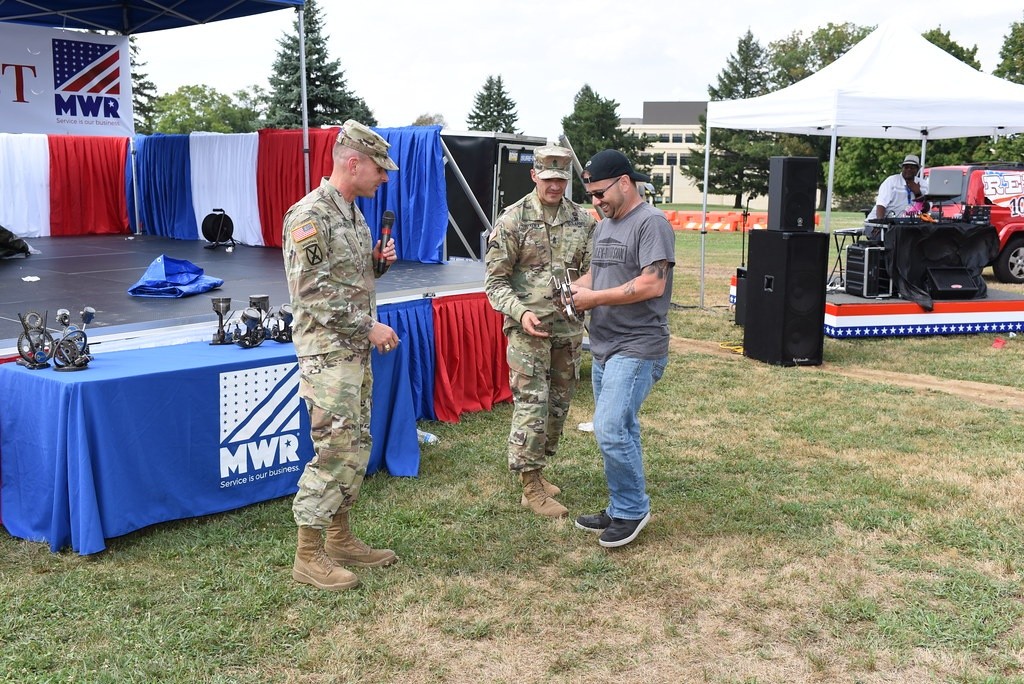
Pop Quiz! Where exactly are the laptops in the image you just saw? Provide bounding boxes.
[912,169,963,202]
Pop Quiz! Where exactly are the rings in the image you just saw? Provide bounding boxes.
[385,344,390,351]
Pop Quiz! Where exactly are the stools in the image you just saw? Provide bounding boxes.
[827,232,862,286]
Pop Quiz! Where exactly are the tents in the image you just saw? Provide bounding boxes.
[700,23,1024,310]
[0,0,310,236]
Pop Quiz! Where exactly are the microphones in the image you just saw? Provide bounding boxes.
[376,210,395,274]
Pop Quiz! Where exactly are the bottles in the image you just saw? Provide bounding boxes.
[415,430,440,444]
[577,421,596,431]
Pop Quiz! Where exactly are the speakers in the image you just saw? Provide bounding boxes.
[735,156,831,369]
[922,267,978,300]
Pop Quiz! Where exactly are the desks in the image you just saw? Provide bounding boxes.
[1,338,420,556]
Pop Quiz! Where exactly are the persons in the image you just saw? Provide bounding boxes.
[561,149,675,546]
[864,155,927,247]
[483,146,598,518]
[282,119,399,590]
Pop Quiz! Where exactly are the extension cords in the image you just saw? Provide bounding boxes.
[826,284,845,292]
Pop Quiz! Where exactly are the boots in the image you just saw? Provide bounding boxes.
[521,469,570,517]
[292,527,358,590]
[324,512,396,568]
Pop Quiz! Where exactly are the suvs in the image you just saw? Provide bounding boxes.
[920,162,1024,284]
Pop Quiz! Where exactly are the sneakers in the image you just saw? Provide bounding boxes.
[599,511,651,548]
[575,509,611,533]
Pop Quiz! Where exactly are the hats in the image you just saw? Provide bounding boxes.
[533,145,572,180]
[901,154,919,171]
[581,149,651,183]
[336,120,399,170]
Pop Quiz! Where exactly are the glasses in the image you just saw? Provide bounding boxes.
[585,177,620,200]
